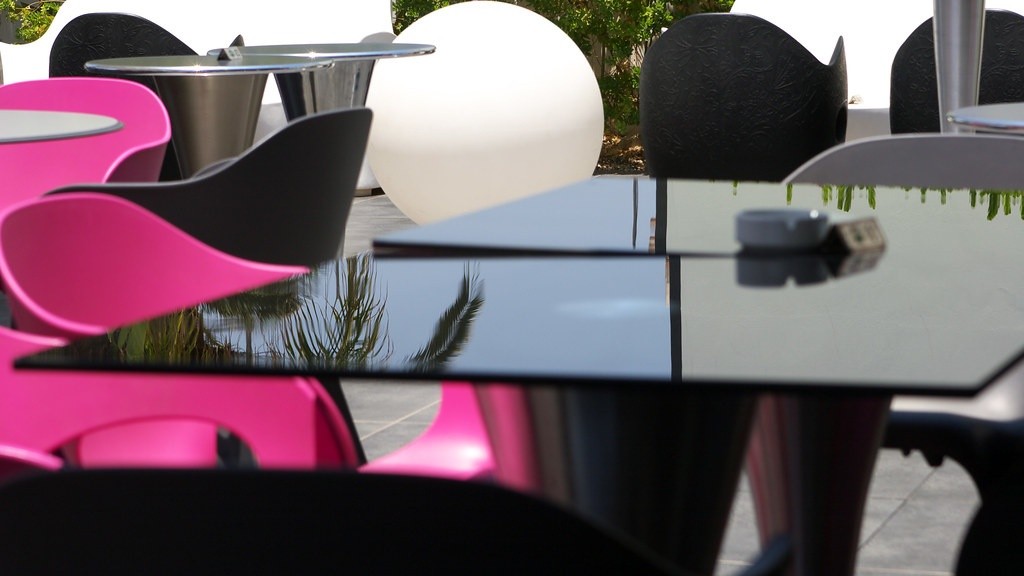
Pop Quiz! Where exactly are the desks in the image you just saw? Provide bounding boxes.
[84,54,335,182]
[948,102,1024,137]
[1,109,126,143]
[206,42,436,123]
[371,172,1024,576]
[14,260,1024,576]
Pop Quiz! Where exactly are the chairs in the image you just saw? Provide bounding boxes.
[0,0,1024,576]
